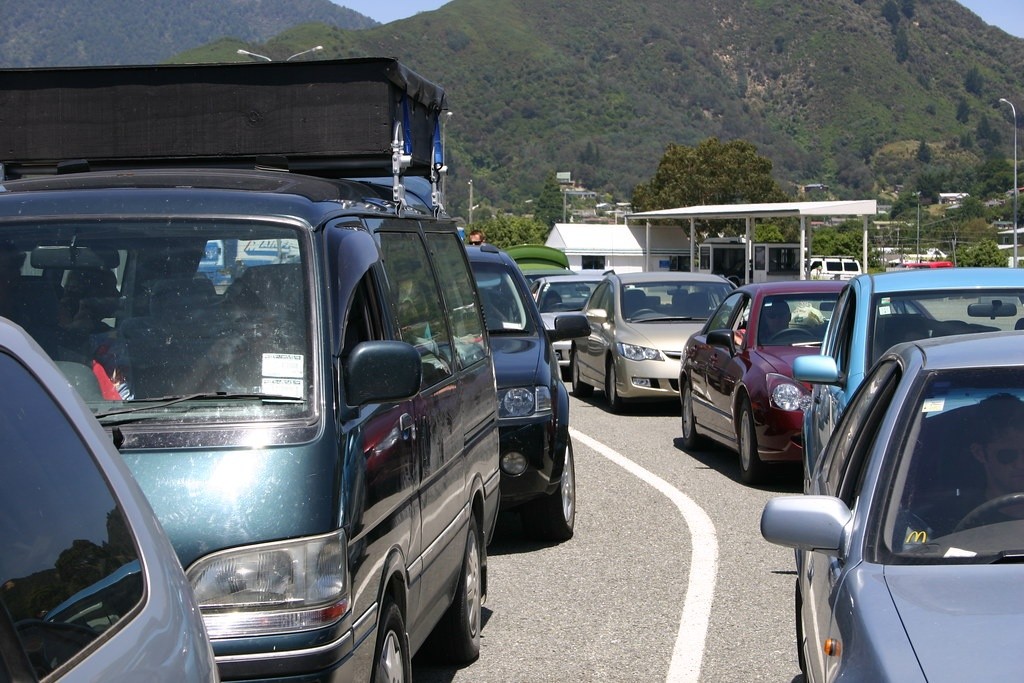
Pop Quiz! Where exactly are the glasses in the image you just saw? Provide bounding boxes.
[767,310,787,319]
[986,445,1022,462]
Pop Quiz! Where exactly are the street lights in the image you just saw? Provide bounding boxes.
[999,98,1018,269]
[441,112,452,205]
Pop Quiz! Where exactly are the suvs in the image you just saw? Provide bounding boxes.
[460,242,591,539]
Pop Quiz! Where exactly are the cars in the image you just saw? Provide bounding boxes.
[678,279,941,486]
[568,269,752,412]
[761,329,1024,683]
[195,174,956,372]
[0,315,222,683]
[791,266,1024,501]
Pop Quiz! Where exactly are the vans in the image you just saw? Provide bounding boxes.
[0,163,504,681]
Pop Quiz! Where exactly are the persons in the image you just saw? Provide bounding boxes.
[178,297,304,399]
[936,392,1024,540]
[759,300,791,344]
[469,229,484,245]
[546,290,558,308]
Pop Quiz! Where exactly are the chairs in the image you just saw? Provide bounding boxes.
[169,262,306,394]
[120,277,225,396]
[689,292,714,317]
[624,289,646,318]
[877,313,930,353]
